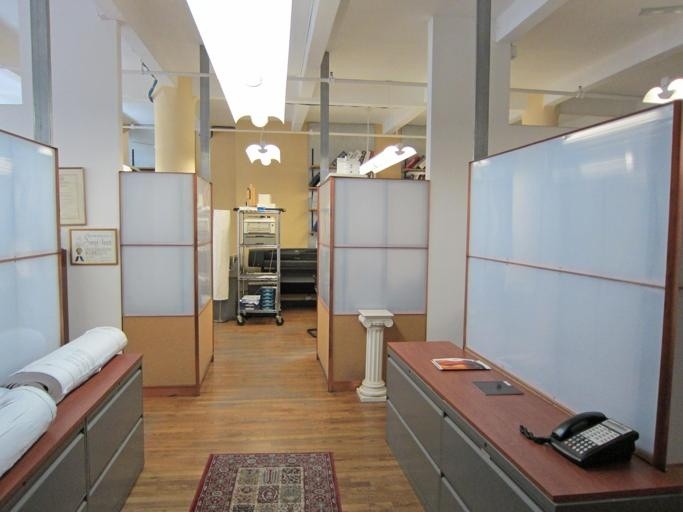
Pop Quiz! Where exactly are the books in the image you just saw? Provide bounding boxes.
[432,357,492,371]
[405,155,426,180]
[472,381,522,396]
[308,151,371,187]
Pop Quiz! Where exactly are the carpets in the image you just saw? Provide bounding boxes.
[189,453,343,512]
[307,328,318,338]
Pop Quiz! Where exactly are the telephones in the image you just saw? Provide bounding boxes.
[545,412,640,469]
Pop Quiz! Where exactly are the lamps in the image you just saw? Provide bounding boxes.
[357,112,416,177]
[243,116,279,168]
[187,0,294,124]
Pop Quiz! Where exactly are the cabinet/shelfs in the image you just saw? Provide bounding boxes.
[384,339,683,512]
[0,351,145,512]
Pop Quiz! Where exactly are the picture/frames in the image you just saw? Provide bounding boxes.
[59,166,88,227]
[68,228,118,266]
